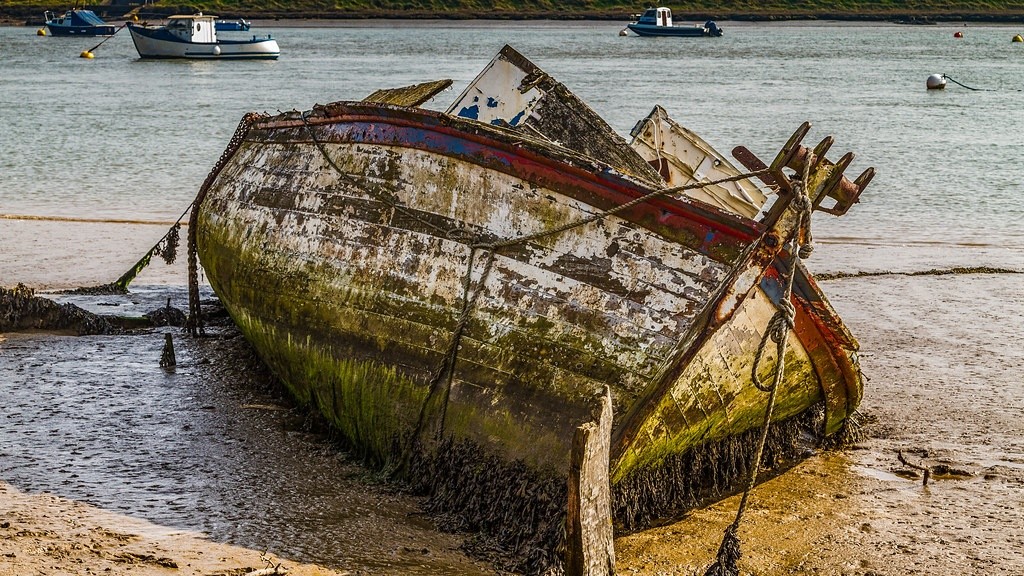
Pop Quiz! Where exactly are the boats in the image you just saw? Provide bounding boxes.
[125,10,280,59]
[43,6,116,37]
[186,44,876,556]
[627,4,724,37]
